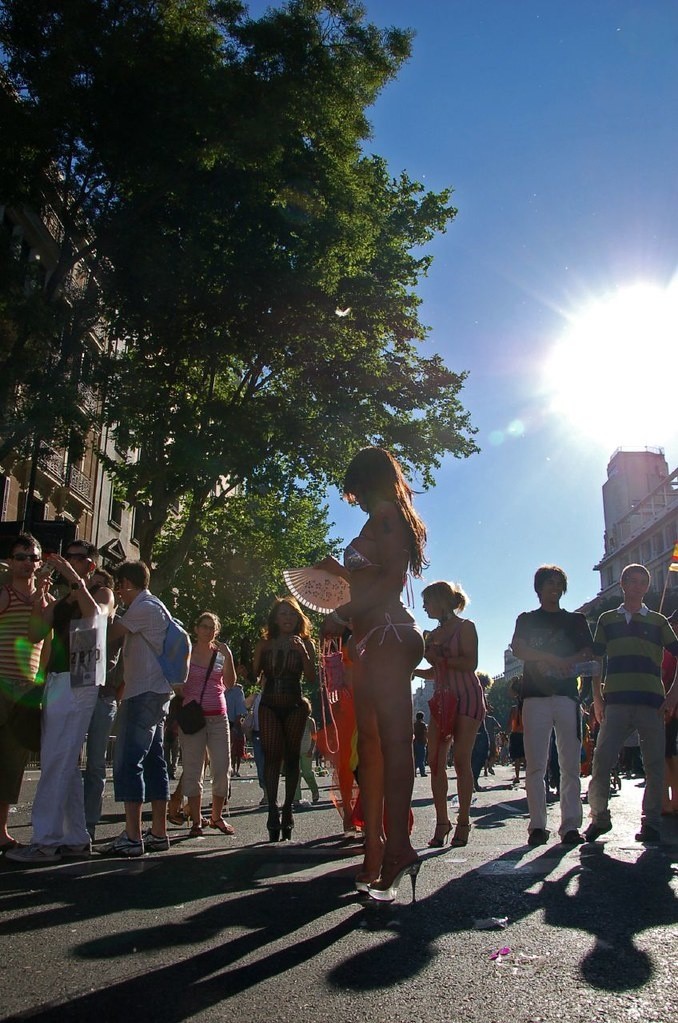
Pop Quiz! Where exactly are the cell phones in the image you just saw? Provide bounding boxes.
[40,561,55,576]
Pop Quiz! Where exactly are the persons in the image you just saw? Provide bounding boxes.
[548,679,646,787]
[0,534,176,863]
[318,622,414,839]
[165,612,319,835]
[414,711,456,777]
[511,569,594,844]
[580,564,678,841]
[662,610,678,816]
[413,583,487,846]
[253,598,316,842]
[314,448,425,902]
[471,674,560,795]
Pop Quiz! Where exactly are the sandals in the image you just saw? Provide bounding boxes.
[190,819,203,836]
[209,818,235,834]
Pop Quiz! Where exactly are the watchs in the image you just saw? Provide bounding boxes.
[71,578,85,591]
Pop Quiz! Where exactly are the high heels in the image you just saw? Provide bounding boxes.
[451,819,471,847]
[428,821,453,847]
[355,865,383,893]
[267,806,281,843]
[182,805,209,827]
[282,808,295,840]
[366,858,423,903]
[167,792,185,824]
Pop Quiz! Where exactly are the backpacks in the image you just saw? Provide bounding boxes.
[124,599,192,685]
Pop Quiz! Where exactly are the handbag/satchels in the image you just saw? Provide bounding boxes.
[242,706,254,739]
[177,700,206,735]
[69,614,107,688]
[429,656,457,740]
[11,684,42,751]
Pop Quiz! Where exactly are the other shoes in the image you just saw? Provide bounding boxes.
[528,829,552,846]
[562,831,585,845]
[292,801,300,809]
[585,822,612,841]
[261,796,266,805]
[313,794,319,803]
[634,826,661,841]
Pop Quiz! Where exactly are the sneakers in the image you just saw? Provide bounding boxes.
[94,830,144,857]
[143,828,170,850]
[62,843,92,857]
[4,844,62,861]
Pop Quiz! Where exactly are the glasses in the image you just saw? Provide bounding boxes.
[64,553,89,560]
[10,554,40,561]
